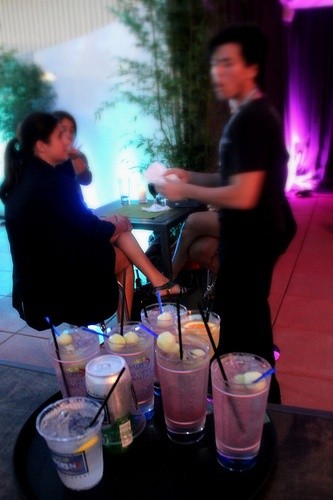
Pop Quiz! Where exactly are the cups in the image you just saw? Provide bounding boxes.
[140,303,189,335]
[48,331,101,397]
[156,335,211,435]
[138,182,147,204]
[36,397,104,490]
[174,309,220,359]
[118,176,130,203]
[84,356,146,446]
[103,323,154,413]
[211,352,272,472]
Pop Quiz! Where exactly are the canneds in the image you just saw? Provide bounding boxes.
[85,355,138,453]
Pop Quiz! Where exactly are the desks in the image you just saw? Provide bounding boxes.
[91,198,207,282]
[12,382,333,500]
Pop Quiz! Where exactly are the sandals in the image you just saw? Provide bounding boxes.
[155,279,198,303]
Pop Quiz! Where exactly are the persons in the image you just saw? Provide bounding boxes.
[155,27,298,403]
[55,110,92,184]
[0,113,187,329]
[173,211,219,299]
